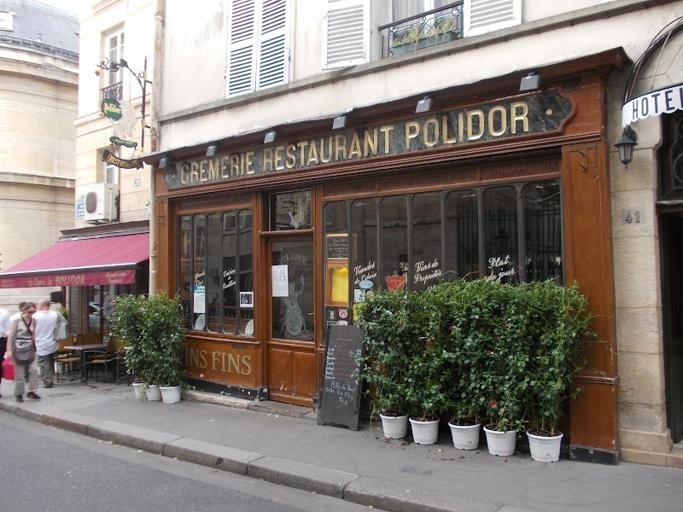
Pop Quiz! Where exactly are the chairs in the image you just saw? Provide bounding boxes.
[46,338,133,384]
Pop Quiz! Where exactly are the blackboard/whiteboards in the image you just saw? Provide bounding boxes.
[317,325,364,431]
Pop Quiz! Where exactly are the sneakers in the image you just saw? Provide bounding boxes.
[16,395,23,402]
[27,392,41,399]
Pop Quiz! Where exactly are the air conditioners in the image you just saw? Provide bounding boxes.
[79,180,121,225]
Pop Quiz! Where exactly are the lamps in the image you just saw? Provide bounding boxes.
[332,115,348,130]
[414,95,434,114]
[205,145,217,156]
[520,72,541,92]
[158,154,169,168]
[615,116,638,173]
[264,131,278,144]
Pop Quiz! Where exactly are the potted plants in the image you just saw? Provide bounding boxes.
[103,290,185,408]
[389,18,458,56]
[351,279,596,463]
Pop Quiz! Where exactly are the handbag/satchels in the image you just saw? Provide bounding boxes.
[54,321,70,342]
[3,359,15,380]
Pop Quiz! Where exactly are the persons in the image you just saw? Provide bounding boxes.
[30,298,68,388]
[6,301,26,323]
[3,300,40,402]
[0,306,13,398]
